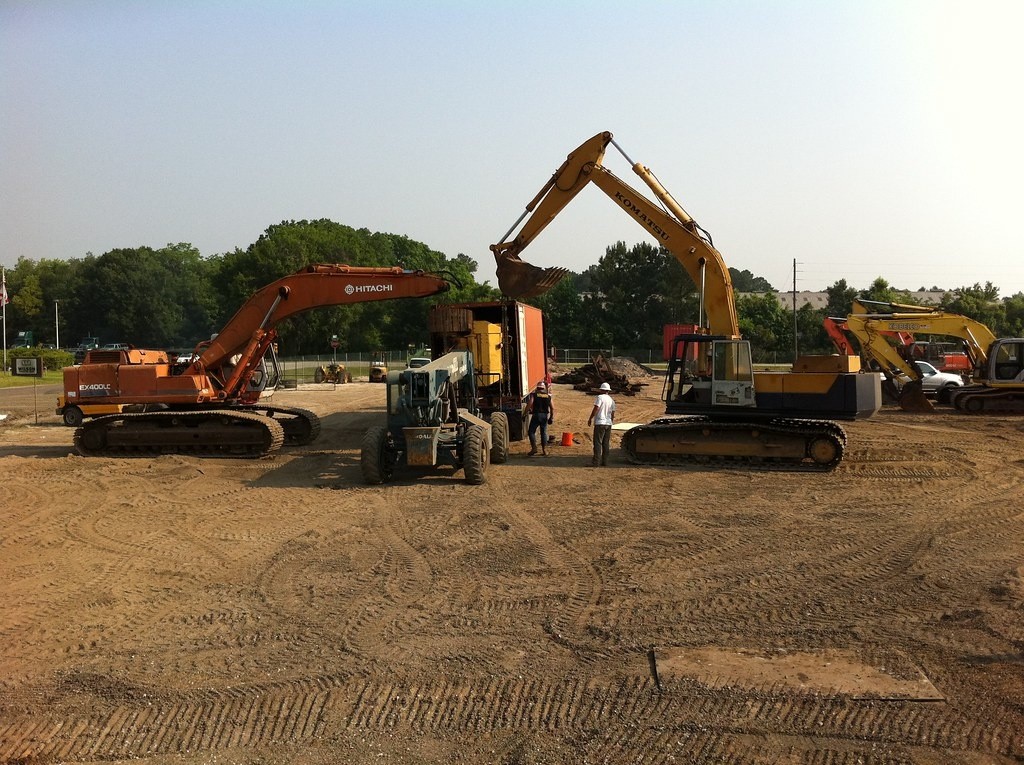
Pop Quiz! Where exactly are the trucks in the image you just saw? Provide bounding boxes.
[11,330,34,350]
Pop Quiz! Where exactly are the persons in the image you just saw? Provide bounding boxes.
[522,382,554,456]
[584,382,616,467]
[406,364,410,369]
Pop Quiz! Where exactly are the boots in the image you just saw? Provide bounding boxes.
[542,445,550,455]
[528,443,538,455]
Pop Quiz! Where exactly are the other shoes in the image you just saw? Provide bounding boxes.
[586,462,599,467]
[602,462,608,466]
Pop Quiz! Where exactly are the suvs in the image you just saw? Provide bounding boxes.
[63,262,466,461]
[101,343,129,351]
[405,358,432,378]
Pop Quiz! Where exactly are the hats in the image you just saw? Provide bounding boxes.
[707,351,713,357]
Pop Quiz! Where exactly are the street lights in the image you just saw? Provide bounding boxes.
[54,299,59,350]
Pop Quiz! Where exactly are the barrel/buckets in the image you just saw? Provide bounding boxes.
[561,431,575,447]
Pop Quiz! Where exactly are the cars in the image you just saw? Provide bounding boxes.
[42,343,56,350]
[879,360,964,393]
[176,352,199,364]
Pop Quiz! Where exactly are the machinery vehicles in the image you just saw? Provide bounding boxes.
[314,362,353,385]
[823,314,973,373]
[489,131,883,471]
[359,301,549,487]
[847,295,1024,415]
[368,351,390,383]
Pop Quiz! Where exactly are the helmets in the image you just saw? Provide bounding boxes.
[599,382,611,391]
[537,381,545,388]
